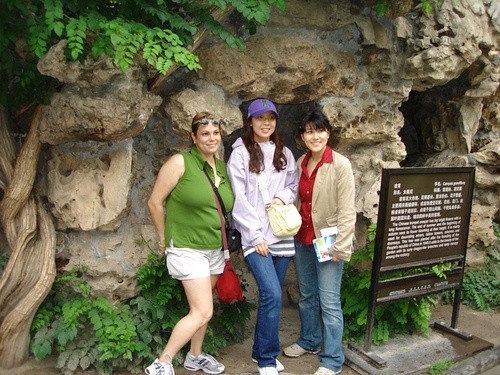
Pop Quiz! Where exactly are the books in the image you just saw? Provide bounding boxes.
[313,226,341,263]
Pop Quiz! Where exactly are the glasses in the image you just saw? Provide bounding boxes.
[193,119,219,126]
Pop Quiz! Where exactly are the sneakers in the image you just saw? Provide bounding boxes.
[313,366,341,375]
[282,343,319,357]
[183,351,225,374]
[144,353,174,375]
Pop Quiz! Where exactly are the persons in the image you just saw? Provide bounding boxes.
[226,98,298,375]
[283,111,356,375]
[144,111,235,375]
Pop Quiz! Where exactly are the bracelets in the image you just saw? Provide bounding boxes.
[157,240,166,248]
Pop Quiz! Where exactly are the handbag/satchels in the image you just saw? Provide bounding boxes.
[217,263,242,305]
[268,204,303,238]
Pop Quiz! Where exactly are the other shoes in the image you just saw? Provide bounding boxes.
[252,358,284,372]
[257,366,278,375]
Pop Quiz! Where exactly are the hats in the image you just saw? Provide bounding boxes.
[247,99,279,119]
